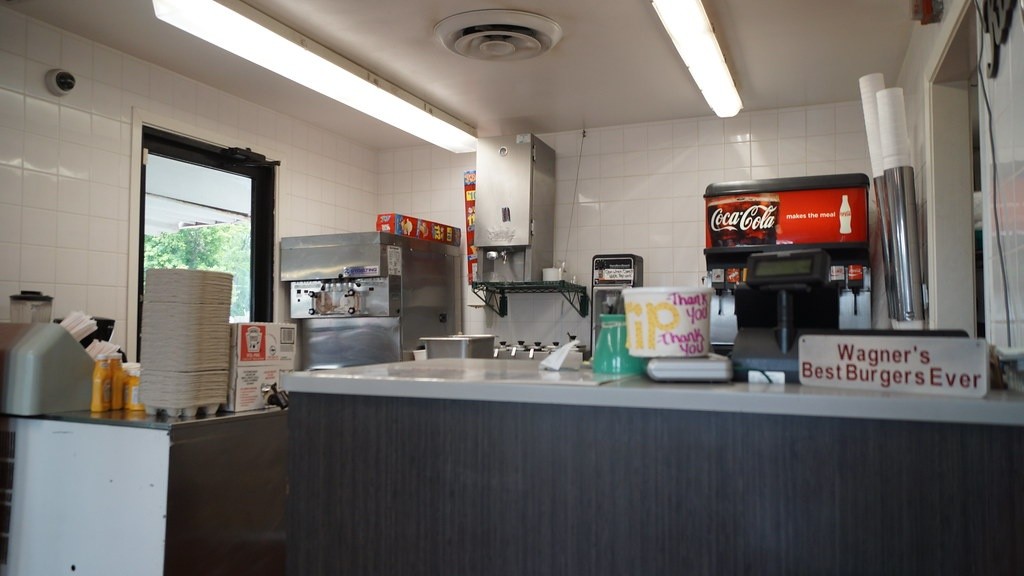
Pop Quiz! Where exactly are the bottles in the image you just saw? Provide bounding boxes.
[92,353,145,412]
[839,195,852,234]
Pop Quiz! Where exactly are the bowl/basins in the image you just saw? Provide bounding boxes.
[623,287,713,358]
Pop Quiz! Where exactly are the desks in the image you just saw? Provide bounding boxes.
[279,358,1024,576]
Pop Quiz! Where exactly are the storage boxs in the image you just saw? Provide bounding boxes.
[543,268,571,281]
[419,335,498,358]
[220,323,297,412]
[412,349,426,360]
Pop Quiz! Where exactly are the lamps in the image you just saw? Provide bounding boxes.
[152,0,478,153]
[651,0,744,118]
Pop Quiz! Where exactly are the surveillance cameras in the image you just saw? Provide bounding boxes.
[45,68,75,97]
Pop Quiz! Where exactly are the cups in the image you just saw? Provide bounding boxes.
[859,71,910,176]
[708,194,780,247]
[543,268,559,281]
[891,319,923,330]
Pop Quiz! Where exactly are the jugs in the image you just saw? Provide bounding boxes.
[9,290,54,323]
